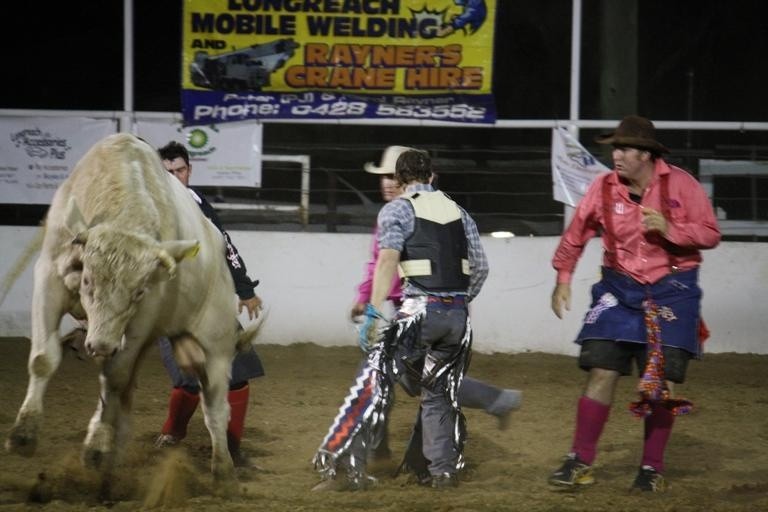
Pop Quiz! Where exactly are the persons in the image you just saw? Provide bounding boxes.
[311,150,487,492]
[548,114,722,492]
[346,143,524,479]
[150,141,270,468]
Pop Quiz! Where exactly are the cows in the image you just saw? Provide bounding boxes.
[4,130,273,499]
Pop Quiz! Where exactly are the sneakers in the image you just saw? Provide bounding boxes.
[153,433,180,448]
[548,451,595,487]
[498,391,522,431]
[628,464,665,493]
[366,457,394,474]
[312,471,348,491]
[422,474,459,488]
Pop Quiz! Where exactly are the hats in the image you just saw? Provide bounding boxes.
[598,115,669,152]
[364,145,429,175]
